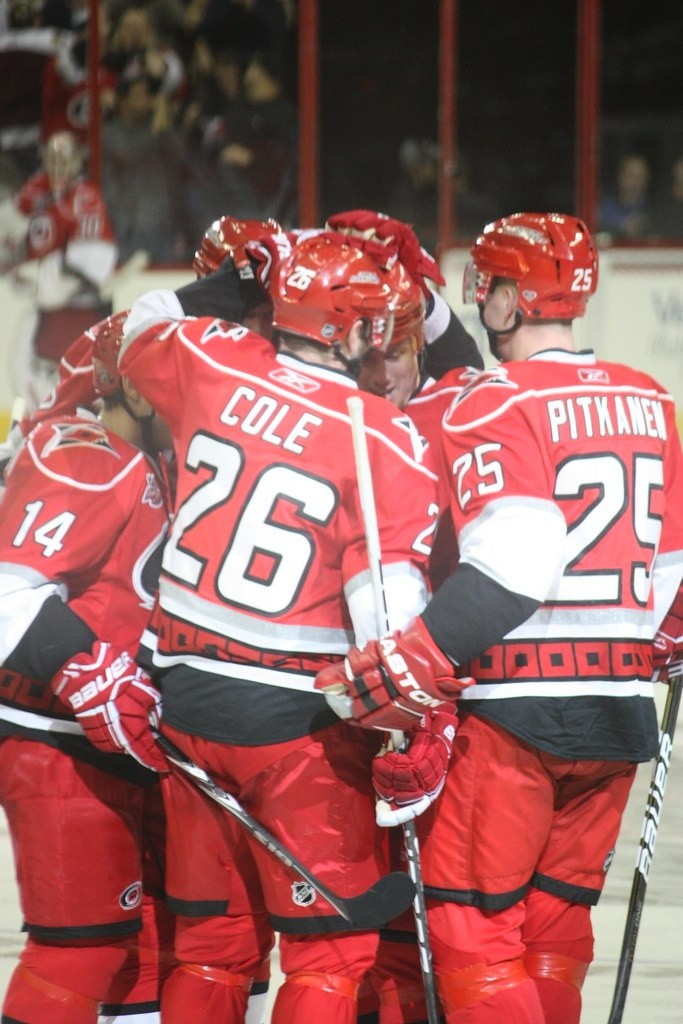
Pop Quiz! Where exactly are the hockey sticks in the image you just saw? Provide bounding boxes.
[347,395,439,1024]
[606,651,683,1024]
[147,725,417,933]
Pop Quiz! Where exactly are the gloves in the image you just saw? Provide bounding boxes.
[219,235,278,315]
[371,701,459,828]
[328,210,446,299]
[51,638,171,774]
[313,617,476,731]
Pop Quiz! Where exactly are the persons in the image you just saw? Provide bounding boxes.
[0,209,485,1024]
[123,234,458,1024]
[314,212,683,1024]
[0,0,683,251]
[0,112,119,436]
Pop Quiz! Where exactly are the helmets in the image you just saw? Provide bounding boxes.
[192,213,277,278]
[377,259,425,355]
[92,309,127,401]
[471,211,599,321]
[271,233,396,354]
